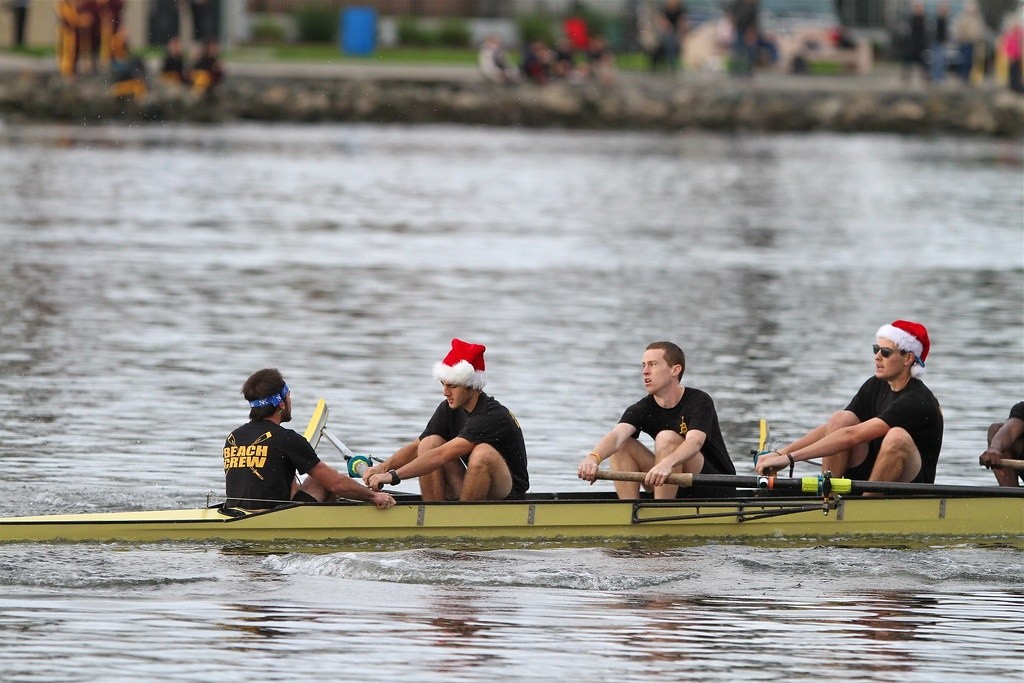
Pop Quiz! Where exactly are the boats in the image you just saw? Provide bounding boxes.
[0,486,1024,544]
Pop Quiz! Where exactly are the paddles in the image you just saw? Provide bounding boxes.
[1000,459,1024,469]
[303,399,384,491]
[759,418,770,489]
[590,468,1024,500]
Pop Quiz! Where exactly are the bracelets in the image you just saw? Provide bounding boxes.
[590,453,601,465]
[787,453,793,462]
[386,468,401,486]
[776,452,781,456]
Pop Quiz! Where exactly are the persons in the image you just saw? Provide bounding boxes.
[362,338,530,503]
[105,30,222,101]
[685,20,862,76]
[901,0,1024,94]
[980,401,1024,487]
[51,0,127,75]
[578,342,737,500]
[478,8,616,83]
[755,320,943,498]
[649,0,685,67]
[223,367,398,510]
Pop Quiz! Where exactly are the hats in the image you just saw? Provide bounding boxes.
[435,339,486,389]
[877,321,930,366]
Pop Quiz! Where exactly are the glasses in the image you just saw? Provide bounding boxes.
[873,344,906,357]
